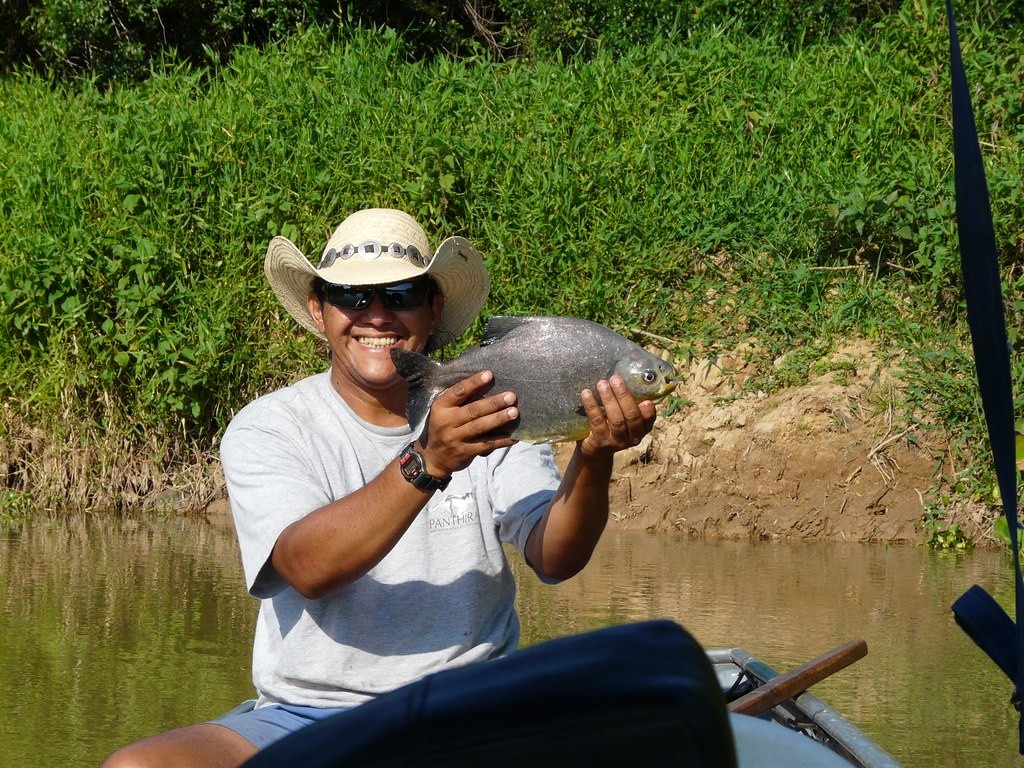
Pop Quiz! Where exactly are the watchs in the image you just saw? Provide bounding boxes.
[400,440,452,492]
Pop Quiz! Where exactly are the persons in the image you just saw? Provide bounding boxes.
[104,209,656,768]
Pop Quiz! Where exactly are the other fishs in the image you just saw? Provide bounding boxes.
[389,315,679,445]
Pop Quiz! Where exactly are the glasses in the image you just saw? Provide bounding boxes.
[312,278,437,312]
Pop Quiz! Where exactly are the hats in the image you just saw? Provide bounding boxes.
[263,209,493,351]
